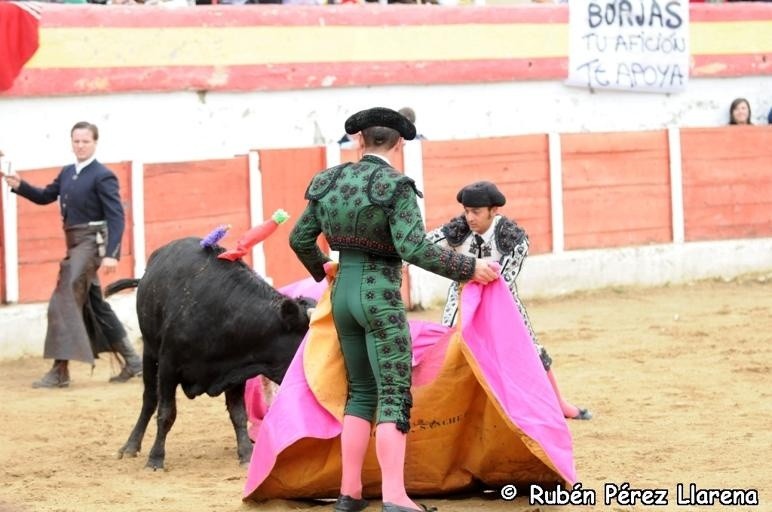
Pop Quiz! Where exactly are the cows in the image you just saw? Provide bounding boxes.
[105,232,319,476]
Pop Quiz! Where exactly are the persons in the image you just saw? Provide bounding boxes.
[1,120,144,389]
[287,106,500,512]
[396,107,427,141]
[401,179,593,420]
[727,98,754,127]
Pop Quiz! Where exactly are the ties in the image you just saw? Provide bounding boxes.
[475,234,483,259]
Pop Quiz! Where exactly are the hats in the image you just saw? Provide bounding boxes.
[457,181,505,207]
[345,107,416,140]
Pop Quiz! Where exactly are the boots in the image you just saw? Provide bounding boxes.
[108,336,143,383]
[31,359,70,388]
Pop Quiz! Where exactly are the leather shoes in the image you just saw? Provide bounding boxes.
[382,502,438,512]
[335,494,369,512]
[561,407,593,419]
